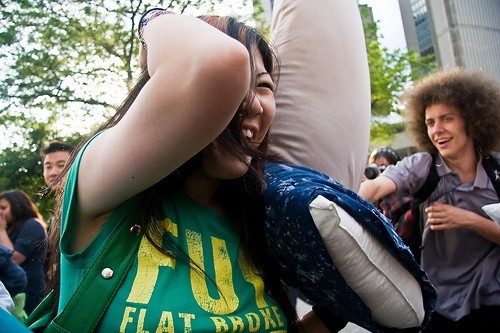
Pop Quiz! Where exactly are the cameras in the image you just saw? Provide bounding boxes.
[365,164,385,180]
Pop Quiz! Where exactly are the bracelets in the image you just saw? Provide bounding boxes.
[137,8,174,48]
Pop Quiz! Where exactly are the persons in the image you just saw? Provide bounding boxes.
[360,146,413,242]
[43,142,73,193]
[357,67,500,333]
[1,190,48,317]
[56,8,350,333]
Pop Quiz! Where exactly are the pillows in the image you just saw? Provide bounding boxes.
[261,162,438,333]
[268,0,372,193]
